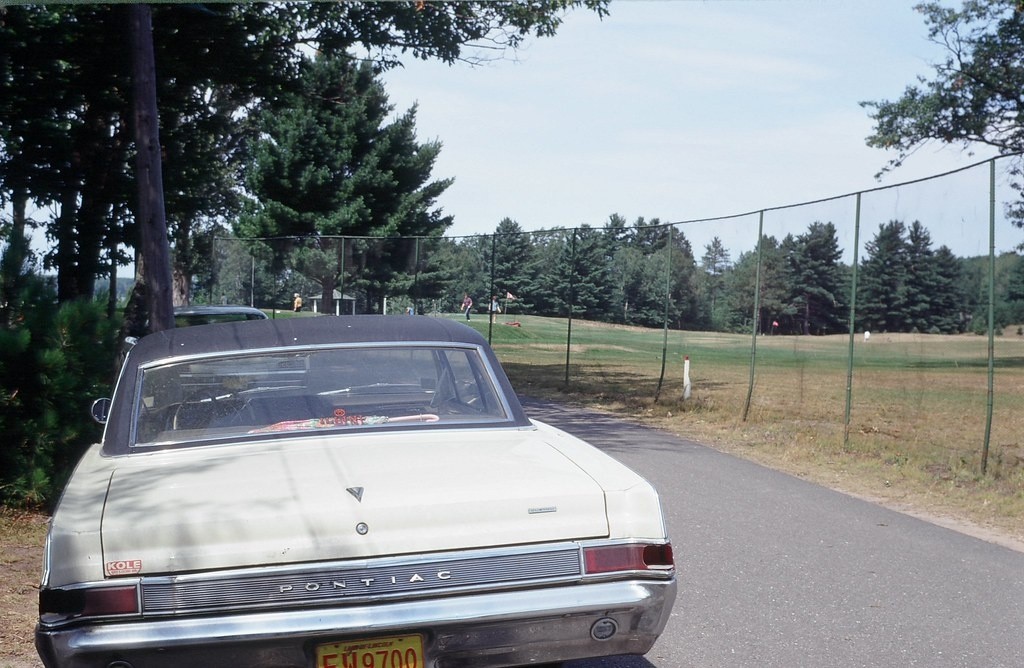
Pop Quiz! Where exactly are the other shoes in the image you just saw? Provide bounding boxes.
[489,320,490,322]
[493,321,496,323]
[468,319,470,322]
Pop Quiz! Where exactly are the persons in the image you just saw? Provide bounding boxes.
[864,331,870,342]
[220,293,228,306]
[405,307,414,315]
[460,293,473,322]
[293,293,302,312]
[488,296,501,323]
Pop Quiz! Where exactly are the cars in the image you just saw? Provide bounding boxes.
[34,315,681,668]
[121,305,310,397]
